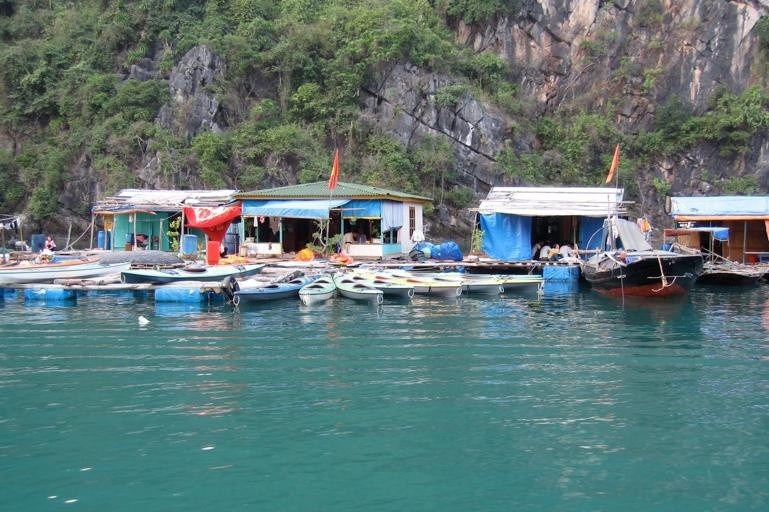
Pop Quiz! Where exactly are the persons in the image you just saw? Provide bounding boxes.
[45,235,56,251]
[532,240,573,261]
[344,231,359,243]
[220,238,229,258]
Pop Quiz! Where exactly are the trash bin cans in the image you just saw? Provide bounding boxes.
[98,231,110,250]
[224,233,239,255]
[663,242,674,252]
[185,234,197,254]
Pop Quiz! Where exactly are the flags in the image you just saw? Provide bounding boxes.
[327,149,339,191]
[605,143,619,184]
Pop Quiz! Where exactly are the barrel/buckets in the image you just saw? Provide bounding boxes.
[225,232,237,254]
[207,241,220,265]
[125,243,132,251]
[125,233,132,242]
[747,253,758,265]
[183,235,197,255]
[543,265,580,295]
[661,243,673,251]
[97,230,108,248]
[15,246,24,251]
